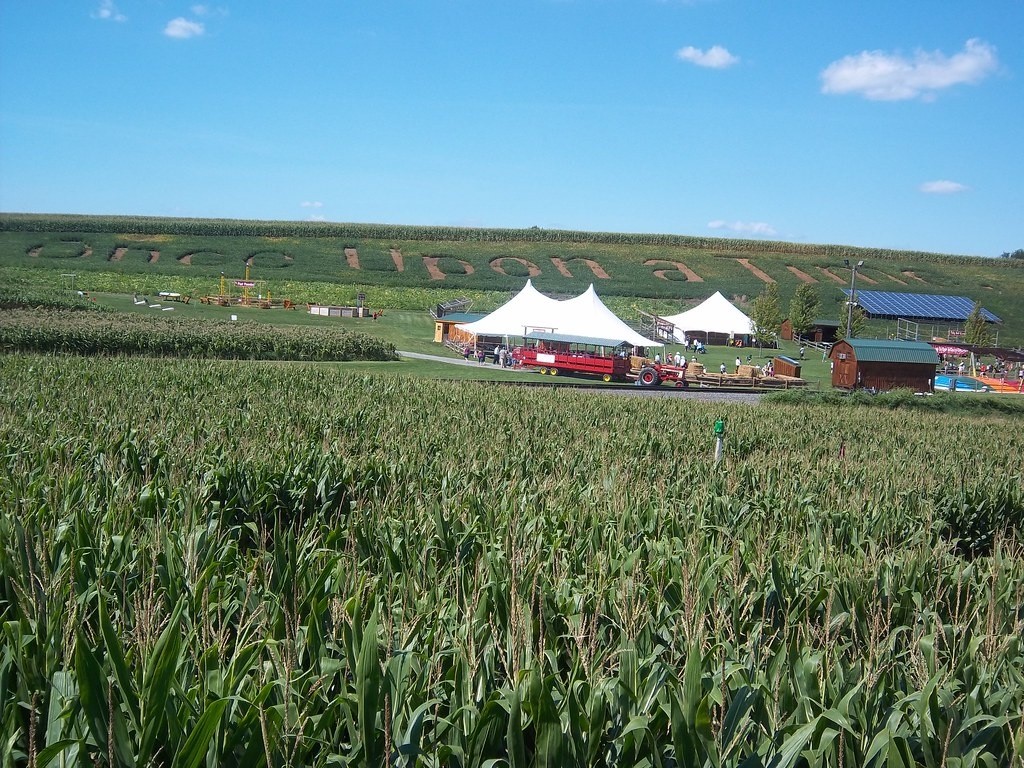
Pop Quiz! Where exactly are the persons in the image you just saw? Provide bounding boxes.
[799,345,805,360]
[830,361,833,373]
[372,309,383,322]
[461,343,508,369]
[720,362,725,374]
[736,357,741,374]
[746,354,752,366]
[674,351,707,373]
[756,359,773,377]
[822,350,827,363]
[725,337,743,348]
[654,351,673,365]
[943,357,1024,383]
[684,335,707,353]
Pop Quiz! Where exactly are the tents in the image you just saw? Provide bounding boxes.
[455,279,560,358]
[657,291,776,345]
[506,284,664,357]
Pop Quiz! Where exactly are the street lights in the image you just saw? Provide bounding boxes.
[843,259,864,339]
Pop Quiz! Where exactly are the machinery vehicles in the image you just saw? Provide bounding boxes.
[522,331,689,389]
